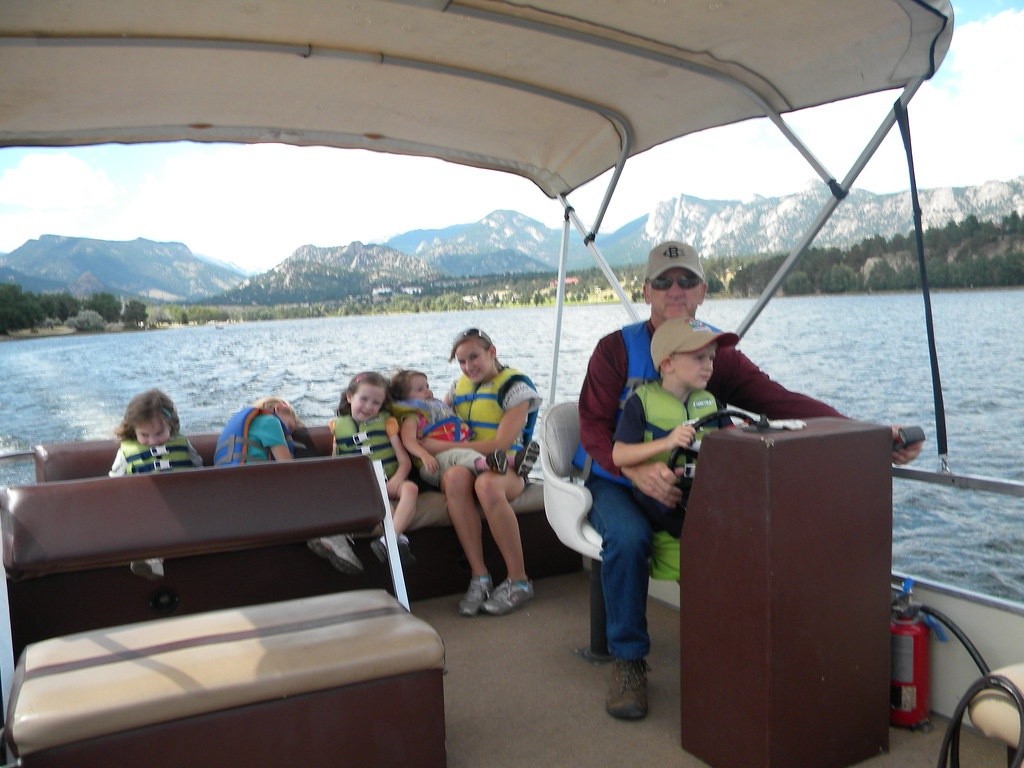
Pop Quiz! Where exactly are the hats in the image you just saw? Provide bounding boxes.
[651,316,738,371]
[646,242,705,286]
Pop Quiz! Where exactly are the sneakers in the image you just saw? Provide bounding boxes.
[482,579,534,614]
[606,656,651,720]
[458,574,491,616]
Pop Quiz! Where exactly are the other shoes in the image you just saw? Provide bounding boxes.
[370,539,387,563]
[398,534,409,547]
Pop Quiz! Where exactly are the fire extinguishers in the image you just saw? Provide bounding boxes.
[890,578,948,725]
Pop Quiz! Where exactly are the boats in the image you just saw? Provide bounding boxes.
[0,1,1023,767]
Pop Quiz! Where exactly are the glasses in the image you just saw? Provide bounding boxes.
[652,272,701,290]
[459,329,491,345]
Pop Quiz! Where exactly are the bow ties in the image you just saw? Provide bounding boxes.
[307,533,364,576]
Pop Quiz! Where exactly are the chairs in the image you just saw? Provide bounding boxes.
[539,399,616,662]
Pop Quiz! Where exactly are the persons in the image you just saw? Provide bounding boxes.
[108,389,202,579]
[389,370,540,488]
[328,372,418,564]
[442,329,543,615]
[213,400,362,575]
[612,316,740,581]
[572,241,924,719]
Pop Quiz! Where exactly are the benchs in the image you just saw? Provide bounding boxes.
[32,410,579,651]
[0,454,449,768]
